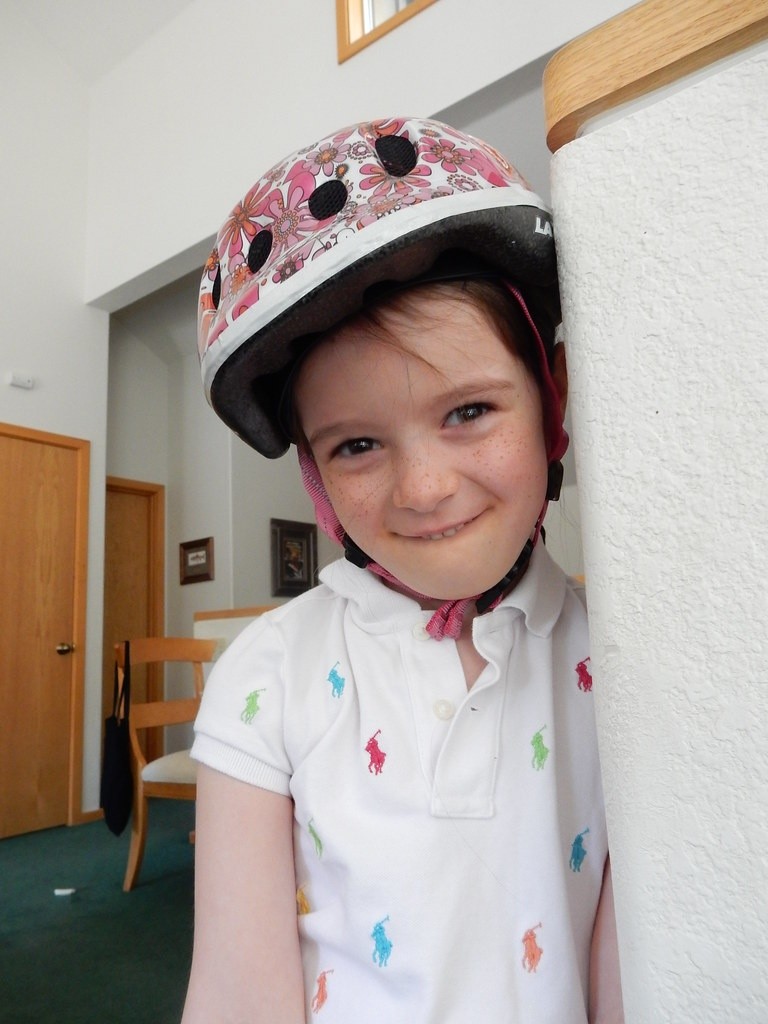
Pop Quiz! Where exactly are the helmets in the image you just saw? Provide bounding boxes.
[195,119,554,459]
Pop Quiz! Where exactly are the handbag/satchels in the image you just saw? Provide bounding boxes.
[100,639,133,838]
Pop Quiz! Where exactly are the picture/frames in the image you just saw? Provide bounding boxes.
[179,537,213,586]
[270,518,319,598]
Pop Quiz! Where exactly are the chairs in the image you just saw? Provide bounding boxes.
[114,637,217,892]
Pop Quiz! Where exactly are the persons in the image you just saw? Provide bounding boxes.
[180,117,623,1024]
